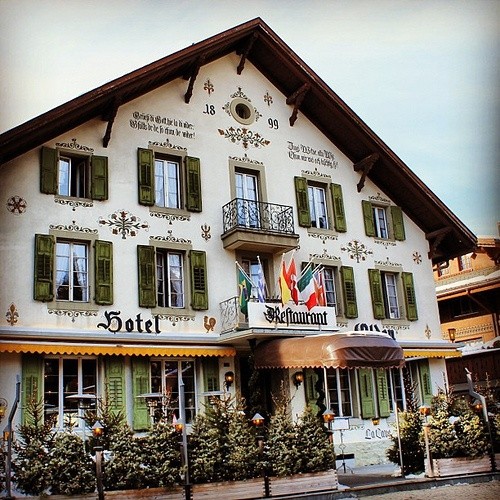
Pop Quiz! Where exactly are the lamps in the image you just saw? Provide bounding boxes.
[175,418,184,442]
[448,327,457,343]
[473,398,483,414]
[0,402,5,423]
[293,371,303,390]
[224,371,234,391]
[91,421,104,445]
[372,415,380,425]
[420,403,430,422]
[2,424,14,441]
[251,413,264,435]
[322,409,335,430]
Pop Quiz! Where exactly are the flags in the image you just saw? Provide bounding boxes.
[277,258,327,311]
[238,265,267,317]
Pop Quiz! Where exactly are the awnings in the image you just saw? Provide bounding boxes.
[250,331,407,370]
